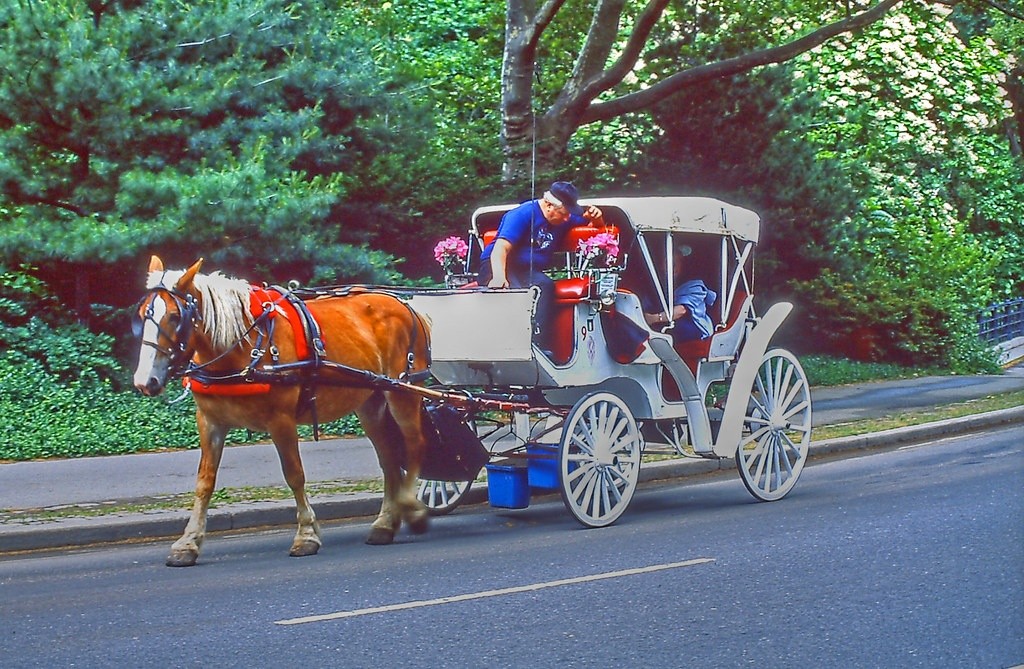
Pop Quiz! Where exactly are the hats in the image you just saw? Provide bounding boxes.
[549,181,584,216]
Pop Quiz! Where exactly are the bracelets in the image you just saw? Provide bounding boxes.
[658,312,662,323]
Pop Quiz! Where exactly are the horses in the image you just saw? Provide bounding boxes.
[133,254,431,567]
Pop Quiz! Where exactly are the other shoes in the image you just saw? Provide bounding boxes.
[540,349,554,360]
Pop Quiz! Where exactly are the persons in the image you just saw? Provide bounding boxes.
[617,251,717,345]
[475,180,610,362]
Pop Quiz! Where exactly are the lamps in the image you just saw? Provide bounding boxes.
[591,268,620,312]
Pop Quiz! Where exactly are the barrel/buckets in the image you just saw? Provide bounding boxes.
[525,445,561,492]
[485,463,529,510]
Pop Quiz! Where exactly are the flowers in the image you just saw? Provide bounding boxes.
[433,235,468,276]
[577,232,618,268]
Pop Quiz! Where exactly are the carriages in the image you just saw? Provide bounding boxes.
[134,81,812,567]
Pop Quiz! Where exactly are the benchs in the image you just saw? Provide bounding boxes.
[484,226,621,365]
[611,289,756,397]
